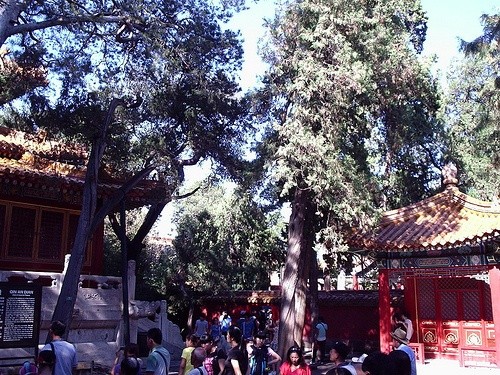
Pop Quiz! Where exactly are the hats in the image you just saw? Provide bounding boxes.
[390,328,408,345]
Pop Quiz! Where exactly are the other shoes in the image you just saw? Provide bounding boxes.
[319,361,323,364]
[310,361,315,365]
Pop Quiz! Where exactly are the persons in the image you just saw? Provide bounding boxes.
[20,350,56,375]
[110,343,144,375]
[119,356,140,375]
[311,316,329,364]
[359,311,416,375]
[178,309,358,375]
[146,327,171,375]
[40,319,78,375]
